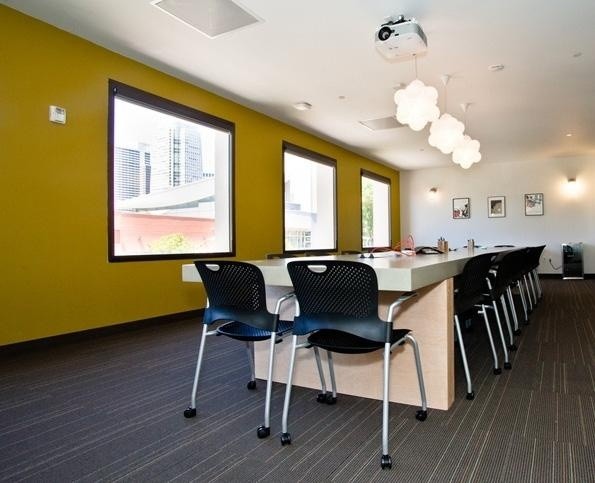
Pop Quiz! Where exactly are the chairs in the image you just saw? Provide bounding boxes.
[281,260,426,468]
[453,243,546,401]
[183,259,325,439]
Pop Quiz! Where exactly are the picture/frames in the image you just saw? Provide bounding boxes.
[452,198,470,219]
[487,196,505,218]
[524,193,544,216]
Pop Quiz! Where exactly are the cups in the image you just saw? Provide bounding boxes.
[438,240,448,254]
[468,239,474,249]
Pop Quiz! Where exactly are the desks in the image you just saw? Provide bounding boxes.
[182,245,533,410]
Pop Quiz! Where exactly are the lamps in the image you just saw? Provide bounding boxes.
[428,74,464,154]
[393,53,438,132]
[452,103,480,168]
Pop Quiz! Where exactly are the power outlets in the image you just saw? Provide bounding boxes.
[547,256,551,262]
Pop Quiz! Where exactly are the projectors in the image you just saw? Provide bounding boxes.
[375,21,428,59]
